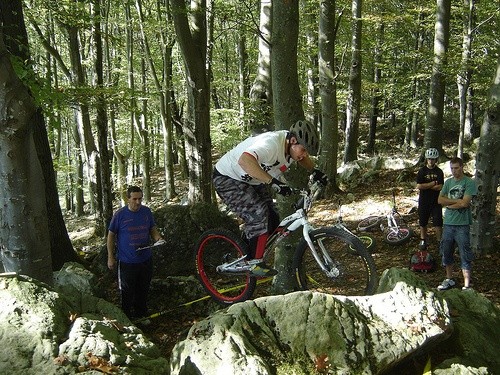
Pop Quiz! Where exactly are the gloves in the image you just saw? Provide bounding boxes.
[268,177,291,198]
[308,169,329,188]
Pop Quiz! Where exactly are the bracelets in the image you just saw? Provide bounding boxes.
[157,237,160,241]
[434,181,436,186]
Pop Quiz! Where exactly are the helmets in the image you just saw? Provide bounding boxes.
[426,148,439,158]
[289,121,318,157]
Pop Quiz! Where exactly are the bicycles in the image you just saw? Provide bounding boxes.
[311,199,378,255]
[193,178,378,307]
[356,191,411,246]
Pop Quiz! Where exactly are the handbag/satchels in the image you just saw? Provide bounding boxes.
[410,251,439,272]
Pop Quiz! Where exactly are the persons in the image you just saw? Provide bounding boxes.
[416,148,444,251]
[437,157,476,291]
[213,120,329,277]
[107,185,166,321]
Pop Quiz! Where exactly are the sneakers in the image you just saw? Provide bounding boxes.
[249,264,277,278]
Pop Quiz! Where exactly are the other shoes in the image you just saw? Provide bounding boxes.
[132,317,150,326]
[419,239,427,250]
[438,278,456,289]
